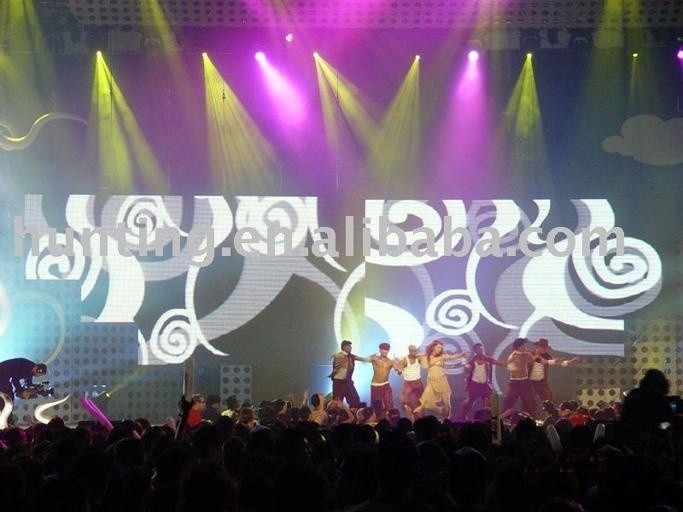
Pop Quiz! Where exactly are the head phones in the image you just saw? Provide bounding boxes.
[37,364,43,373]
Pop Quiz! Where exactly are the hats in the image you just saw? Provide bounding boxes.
[533,339,548,346]
[379,343,391,350]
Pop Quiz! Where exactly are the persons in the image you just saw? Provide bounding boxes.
[0,337,683,512]
